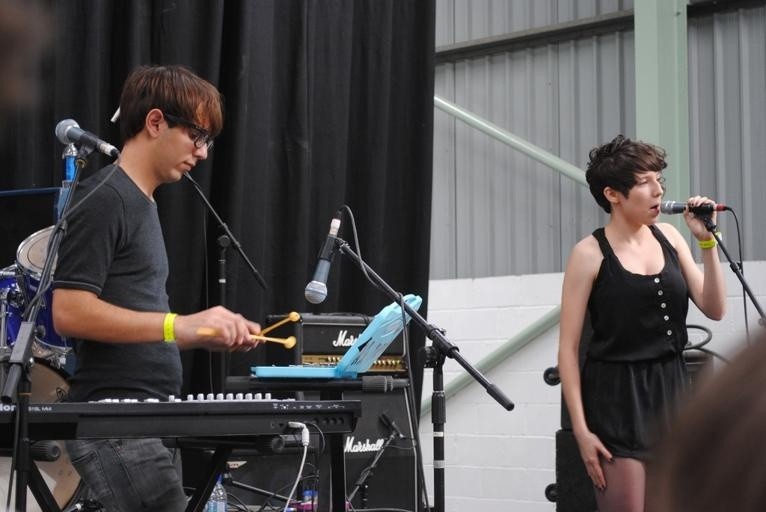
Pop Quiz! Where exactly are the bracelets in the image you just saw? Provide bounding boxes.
[162,312,179,343]
[697,231,723,248]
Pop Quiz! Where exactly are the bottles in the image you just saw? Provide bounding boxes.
[203,474,227,512]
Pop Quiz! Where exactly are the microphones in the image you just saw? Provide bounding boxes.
[660,200,725,214]
[54,118,119,159]
[304,208,344,305]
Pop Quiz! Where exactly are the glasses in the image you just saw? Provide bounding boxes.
[164,112,215,151]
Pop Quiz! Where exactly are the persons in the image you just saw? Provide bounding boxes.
[557,135,728,511]
[50,60,263,511]
[661,328,766,512]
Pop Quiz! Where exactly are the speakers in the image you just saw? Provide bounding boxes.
[556,349,714,512]
[265,312,423,512]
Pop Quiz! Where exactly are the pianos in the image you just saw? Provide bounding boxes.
[2,390,362,442]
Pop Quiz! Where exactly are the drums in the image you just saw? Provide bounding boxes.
[0,223,77,357]
[0,358,87,512]
